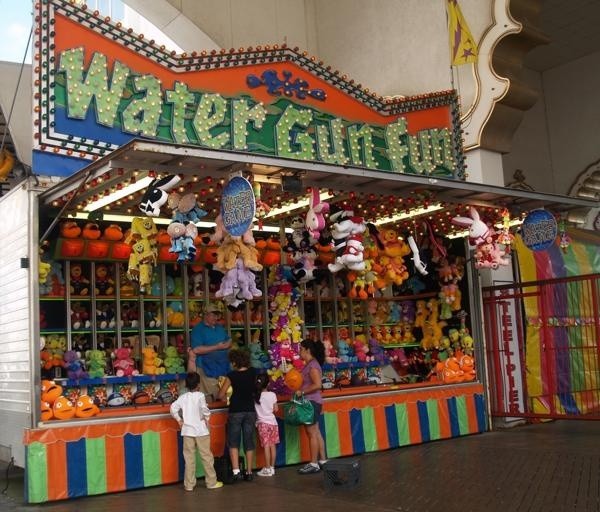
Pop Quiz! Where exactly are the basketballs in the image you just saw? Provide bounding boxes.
[335,374,351,387]
[106,392,124,407]
[352,373,364,385]
[156,388,173,405]
[132,391,150,406]
[366,373,381,385]
[320,376,333,390]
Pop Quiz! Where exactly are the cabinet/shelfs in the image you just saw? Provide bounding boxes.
[38,218,439,411]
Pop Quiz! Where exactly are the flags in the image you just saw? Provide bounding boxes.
[444,1,479,68]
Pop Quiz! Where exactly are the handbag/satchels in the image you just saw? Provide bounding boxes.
[283,399,314,426]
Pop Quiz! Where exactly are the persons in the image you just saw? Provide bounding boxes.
[169,371,224,492]
[217,347,259,484]
[293,338,329,475]
[251,372,281,478]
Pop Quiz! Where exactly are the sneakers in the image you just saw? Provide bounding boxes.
[207,481,223,489]
[227,469,253,482]
[256,464,276,476]
[298,458,329,474]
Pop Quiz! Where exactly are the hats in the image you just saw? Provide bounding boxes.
[206,303,222,313]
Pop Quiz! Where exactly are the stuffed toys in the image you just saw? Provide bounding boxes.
[33,168,517,422]
[39,174,516,422]
